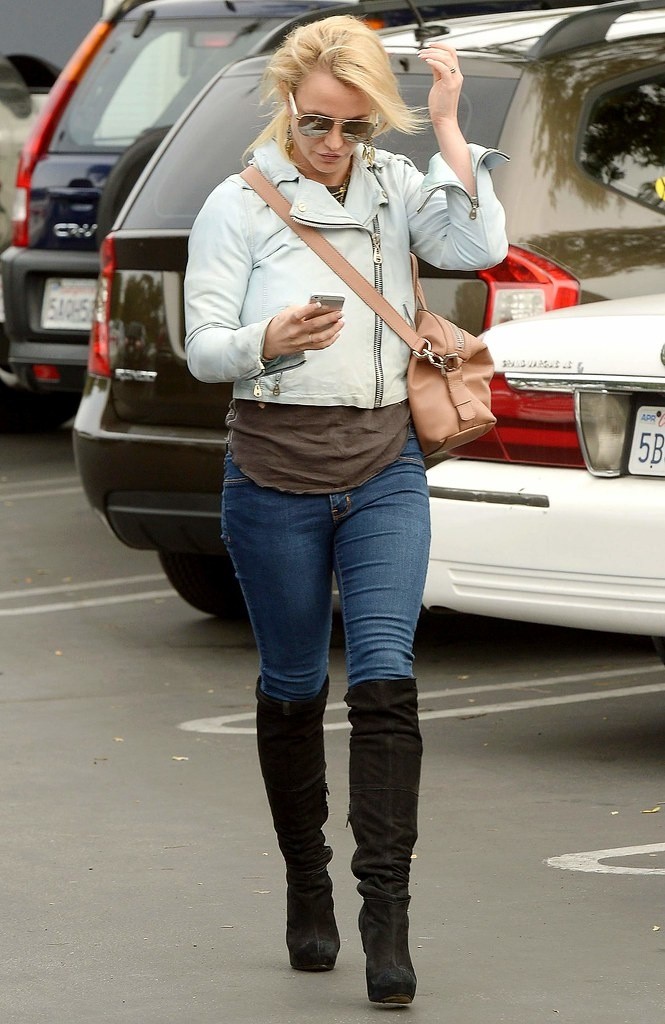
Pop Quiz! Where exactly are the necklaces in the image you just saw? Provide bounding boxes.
[329,166,351,206]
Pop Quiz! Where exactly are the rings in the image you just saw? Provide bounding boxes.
[309,335,312,343]
[450,66,457,73]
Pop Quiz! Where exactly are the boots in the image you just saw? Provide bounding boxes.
[254,674,341,970]
[346,676,424,1006]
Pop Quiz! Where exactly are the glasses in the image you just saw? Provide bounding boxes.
[289,91,378,143]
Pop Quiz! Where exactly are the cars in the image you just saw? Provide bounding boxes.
[330,294,664,641]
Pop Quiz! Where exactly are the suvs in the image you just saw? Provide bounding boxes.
[0,0,232,249]
[69,1,665,626]
[0,0,607,435]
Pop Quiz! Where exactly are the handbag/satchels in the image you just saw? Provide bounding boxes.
[409,308,498,457]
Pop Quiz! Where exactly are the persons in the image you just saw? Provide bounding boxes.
[186,14,509,1008]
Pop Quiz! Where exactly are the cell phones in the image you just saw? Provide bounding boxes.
[304,291,346,321]
